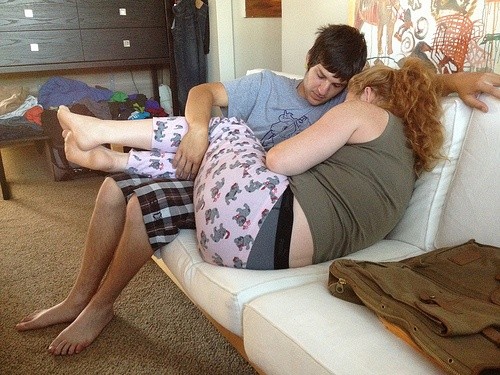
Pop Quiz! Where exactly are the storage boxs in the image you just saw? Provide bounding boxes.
[48,143,112,183]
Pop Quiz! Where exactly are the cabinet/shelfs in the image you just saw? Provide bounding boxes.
[0,0,180,201]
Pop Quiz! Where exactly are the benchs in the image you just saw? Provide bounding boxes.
[111,68,498,375]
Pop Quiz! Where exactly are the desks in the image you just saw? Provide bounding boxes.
[0,106,46,202]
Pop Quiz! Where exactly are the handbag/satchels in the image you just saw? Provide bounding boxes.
[328,238,500,375]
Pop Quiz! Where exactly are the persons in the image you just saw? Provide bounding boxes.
[15,24,500,355]
[56,55,446,270]
